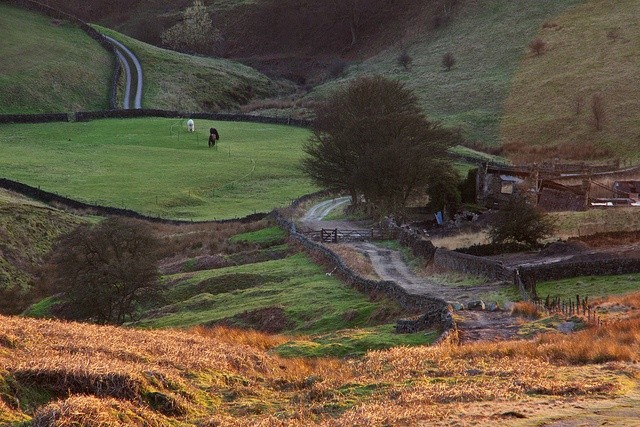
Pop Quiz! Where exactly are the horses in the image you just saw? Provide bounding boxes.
[208,134,216,148]
[210,128,220,140]
[187,119,194,132]
